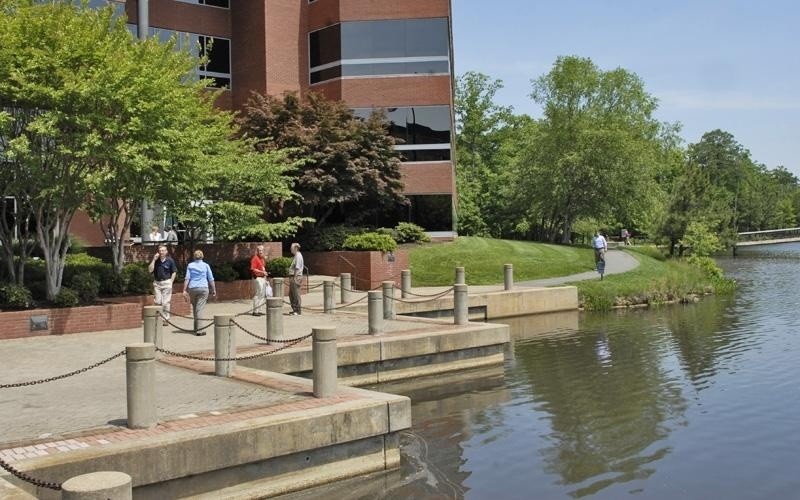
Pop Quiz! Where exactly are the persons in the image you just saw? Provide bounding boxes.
[148,245,178,326]
[250,246,269,316]
[149,226,180,242]
[623,229,631,245]
[592,230,607,271]
[182,250,217,336]
[288,242,303,316]
